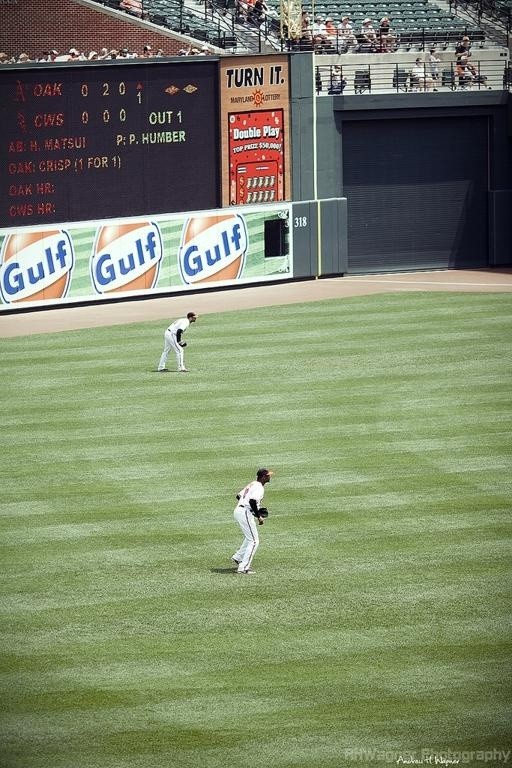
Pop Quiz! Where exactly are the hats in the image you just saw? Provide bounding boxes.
[257,469,274,477]
[187,312,199,318]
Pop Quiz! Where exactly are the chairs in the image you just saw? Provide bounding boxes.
[314,62,457,93]
[137,1,512,54]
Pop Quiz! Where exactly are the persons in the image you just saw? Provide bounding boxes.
[156,311,199,373]
[236,1,397,52]
[454,55,493,90]
[455,37,472,88]
[1,45,211,64]
[429,48,440,90]
[329,64,347,92]
[230,466,275,575]
[412,56,436,92]
